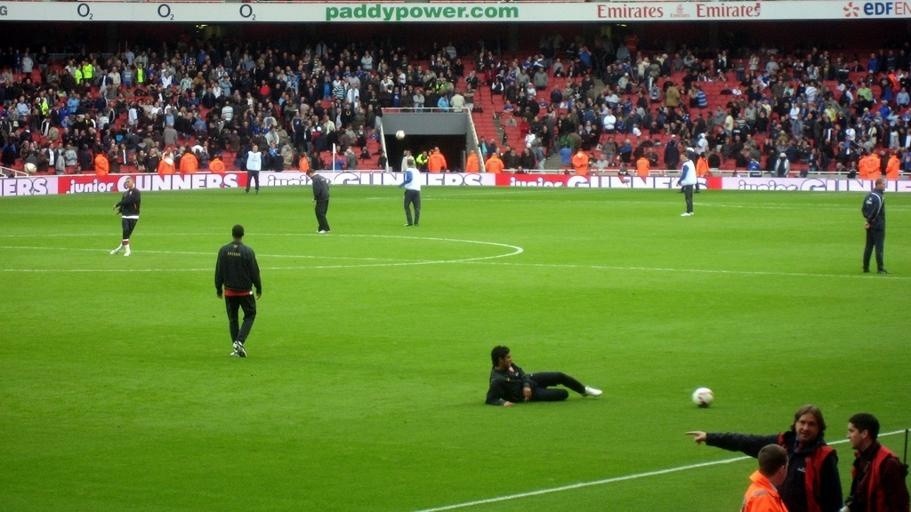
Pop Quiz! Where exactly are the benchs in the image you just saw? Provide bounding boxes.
[0,47,911,183]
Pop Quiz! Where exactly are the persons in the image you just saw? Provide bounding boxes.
[484,344,604,409]
[677,153,698,217]
[243,143,263,194]
[397,157,422,227]
[860,177,891,276]
[838,412,911,512]
[110,177,141,256]
[305,168,332,234]
[738,442,791,511]
[212,224,265,361]
[683,404,844,511]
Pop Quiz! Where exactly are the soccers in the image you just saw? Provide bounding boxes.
[693,387,713,407]
[396,130,405,140]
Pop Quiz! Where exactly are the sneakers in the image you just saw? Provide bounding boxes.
[123,250,131,256]
[229,342,248,358]
[863,268,889,275]
[316,228,330,233]
[109,247,119,255]
[680,211,695,217]
[404,223,419,227]
[581,383,603,397]
